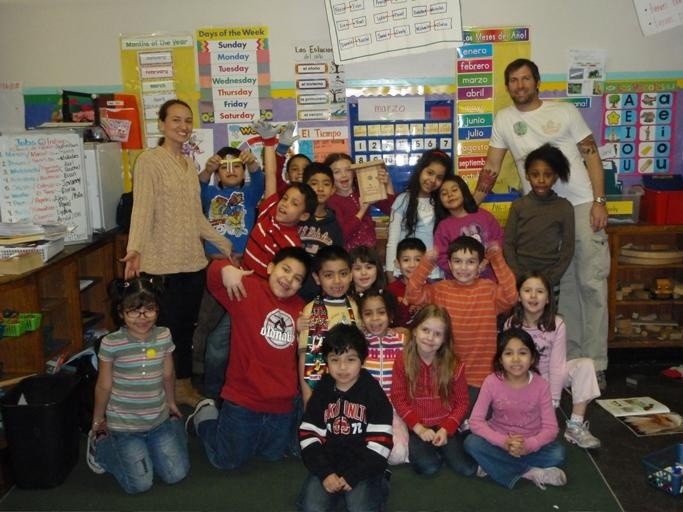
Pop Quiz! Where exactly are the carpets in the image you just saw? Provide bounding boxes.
[555,345,682,511]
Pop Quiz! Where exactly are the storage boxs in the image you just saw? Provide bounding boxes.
[597,184,646,223]
[639,172,681,226]
[467,187,518,228]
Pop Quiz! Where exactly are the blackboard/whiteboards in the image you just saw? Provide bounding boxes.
[0,130,93,246]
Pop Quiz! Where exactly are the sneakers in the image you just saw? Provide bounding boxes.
[185,398,214,436]
[86,428,106,475]
[532,466,567,487]
[174,380,203,407]
[562,418,601,450]
[597,370,606,390]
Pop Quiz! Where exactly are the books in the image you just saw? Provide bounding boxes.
[0,219,79,245]
[597,395,683,437]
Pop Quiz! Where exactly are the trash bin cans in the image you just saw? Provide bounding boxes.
[0,377,80,489]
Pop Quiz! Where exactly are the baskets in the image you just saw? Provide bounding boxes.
[642,445,683,496]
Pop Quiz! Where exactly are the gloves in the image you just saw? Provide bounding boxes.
[252,119,281,145]
[277,122,299,154]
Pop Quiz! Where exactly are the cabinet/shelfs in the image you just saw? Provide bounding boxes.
[76,231,122,352]
[612,226,682,366]
[37,248,78,371]
[0,272,39,387]
